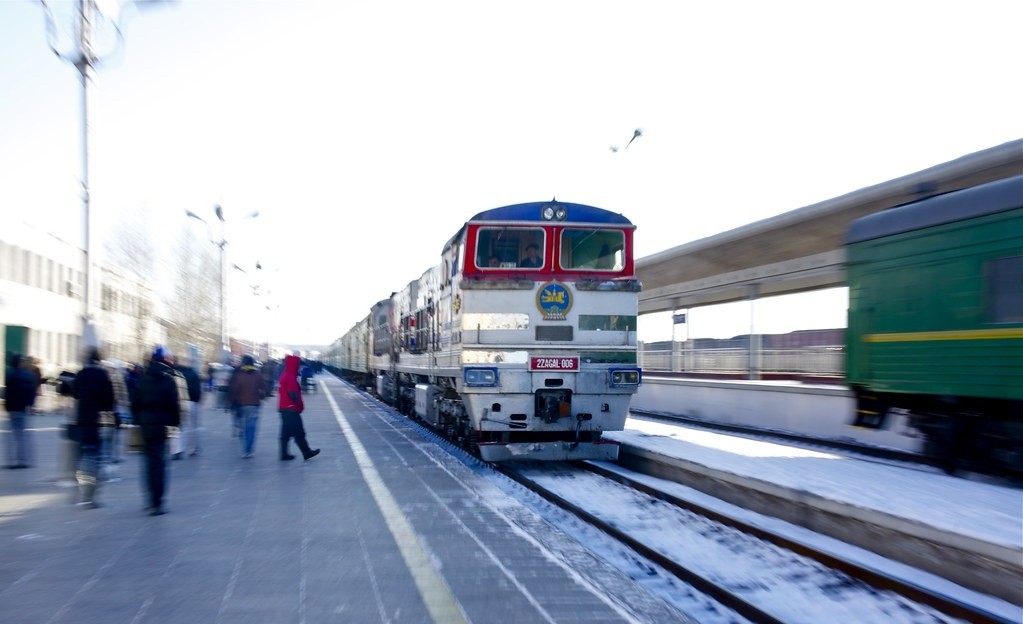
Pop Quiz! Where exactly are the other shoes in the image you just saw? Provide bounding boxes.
[304,449,320,460]
[241,452,254,458]
[282,454,295,461]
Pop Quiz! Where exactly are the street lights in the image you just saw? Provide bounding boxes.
[186,205,259,364]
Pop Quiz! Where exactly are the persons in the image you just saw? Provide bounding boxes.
[277,356,320,460]
[520,244,542,268]
[488,256,500,267]
[299,359,323,385]
[3,346,280,517]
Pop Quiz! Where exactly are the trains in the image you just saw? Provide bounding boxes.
[317,199,641,463]
[841,174,1023,476]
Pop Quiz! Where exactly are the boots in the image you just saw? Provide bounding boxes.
[78,485,97,509]
